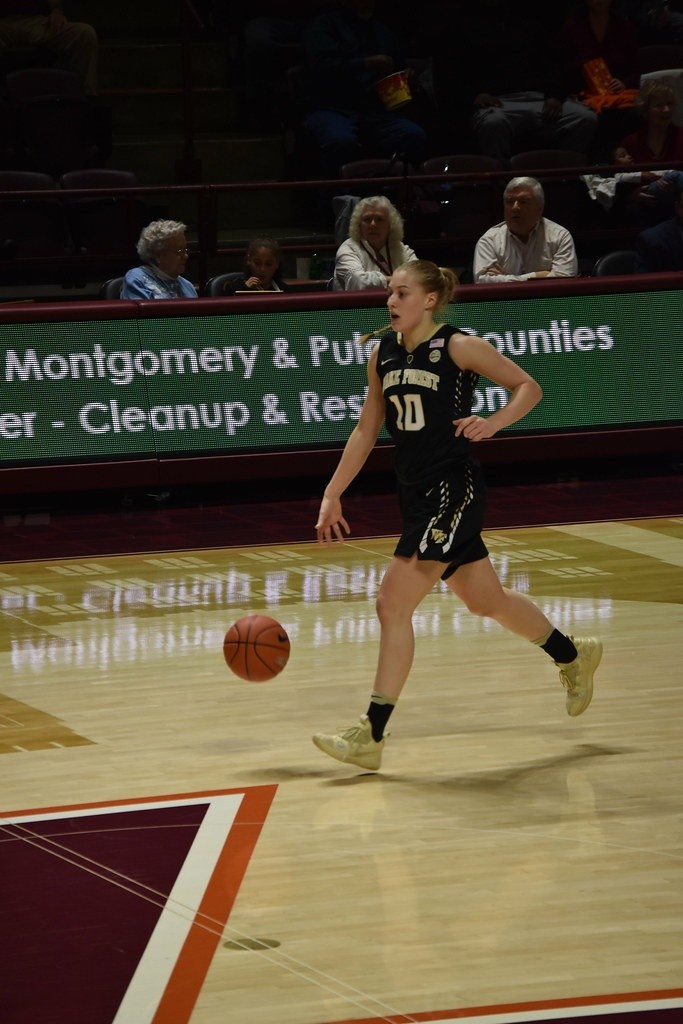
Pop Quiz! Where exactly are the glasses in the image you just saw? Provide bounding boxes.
[173,248,188,256]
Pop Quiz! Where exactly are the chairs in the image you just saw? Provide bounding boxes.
[211,0,683,234]
[0,44,146,285]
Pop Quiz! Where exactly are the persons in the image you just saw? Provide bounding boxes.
[0,0,682,297]
[311,262,604,774]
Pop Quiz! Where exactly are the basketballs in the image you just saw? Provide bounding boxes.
[222,613,290,683]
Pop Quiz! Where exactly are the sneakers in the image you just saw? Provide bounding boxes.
[312,714,390,770]
[551,635,603,716]
